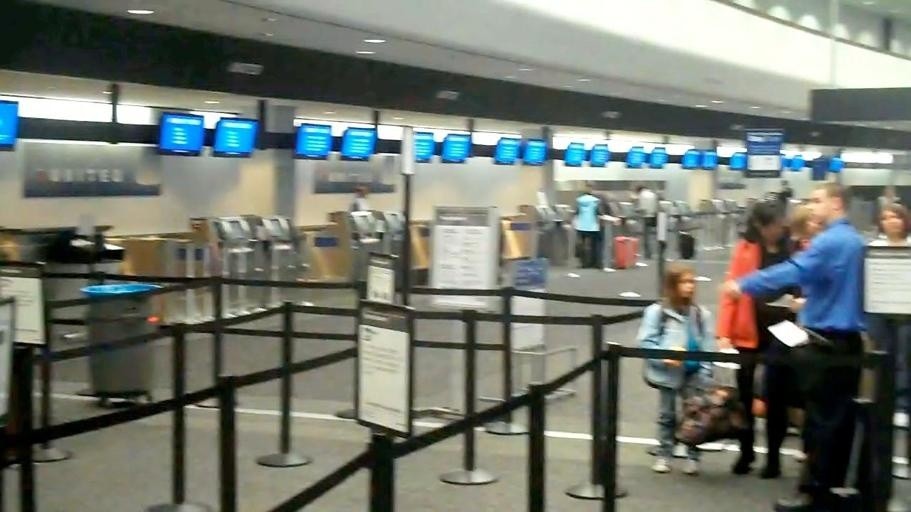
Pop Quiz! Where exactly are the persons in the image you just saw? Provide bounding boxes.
[634,183,658,260]
[570,188,605,269]
[353,184,370,210]
[717,185,911,511]
[635,262,717,477]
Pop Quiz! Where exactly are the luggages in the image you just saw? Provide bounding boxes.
[612,234,640,270]
[679,231,697,261]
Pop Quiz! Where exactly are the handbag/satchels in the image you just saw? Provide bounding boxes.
[673,388,745,445]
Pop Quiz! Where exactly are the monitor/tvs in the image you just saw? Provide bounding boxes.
[564,142,584,166]
[781,157,791,168]
[414,132,434,163]
[341,127,378,161]
[213,117,259,158]
[700,149,717,170]
[650,147,666,168]
[591,144,609,167]
[157,111,204,155]
[442,133,472,163]
[509,135,510,137]
[729,152,746,169]
[523,138,546,166]
[293,123,332,160]
[0,100,20,151]
[790,154,804,171]
[827,156,844,173]
[626,147,646,168]
[495,136,522,165]
[682,148,699,169]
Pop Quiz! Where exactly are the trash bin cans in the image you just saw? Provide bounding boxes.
[679,234,695,258]
[76,283,165,407]
[613,237,639,268]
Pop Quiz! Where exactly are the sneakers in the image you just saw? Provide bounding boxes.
[648,442,892,512]
